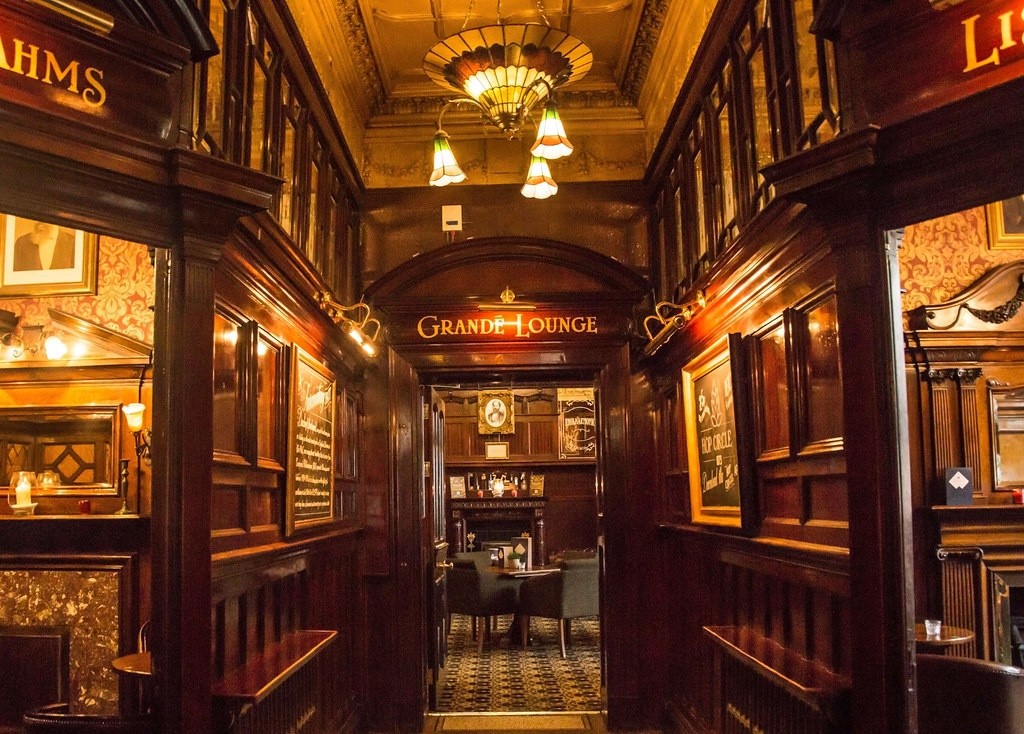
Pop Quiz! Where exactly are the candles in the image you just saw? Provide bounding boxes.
[15,481,32,506]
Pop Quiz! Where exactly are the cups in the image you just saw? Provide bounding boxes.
[925,620,941,635]
[519,563,525,573]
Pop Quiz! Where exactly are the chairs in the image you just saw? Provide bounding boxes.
[446,549,598,659]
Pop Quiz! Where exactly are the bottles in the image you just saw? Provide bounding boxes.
[509,473,529,497]
[472,473,478,485]
[481,474,486,489]
[488,473,509,490]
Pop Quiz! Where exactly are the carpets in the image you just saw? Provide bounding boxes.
[422,611,606,715]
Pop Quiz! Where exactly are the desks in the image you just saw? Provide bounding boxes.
[449,495,549,565]
[486,563,563,643]
[914,622,976,655]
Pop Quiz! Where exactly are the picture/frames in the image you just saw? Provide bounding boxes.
[0,213,100,300]
[680,333,750,528]
[985,193,1024,250]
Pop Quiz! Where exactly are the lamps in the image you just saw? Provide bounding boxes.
[317,290,381,357]
[121,402,152,466]
[642,290,707,357]
[422,0,594,201]
[0,327,89,363]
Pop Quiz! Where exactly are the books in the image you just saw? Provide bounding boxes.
[498,545,514,569]
[512,537,532,570]
[450,477,466,498]
[530,475,545,497]
